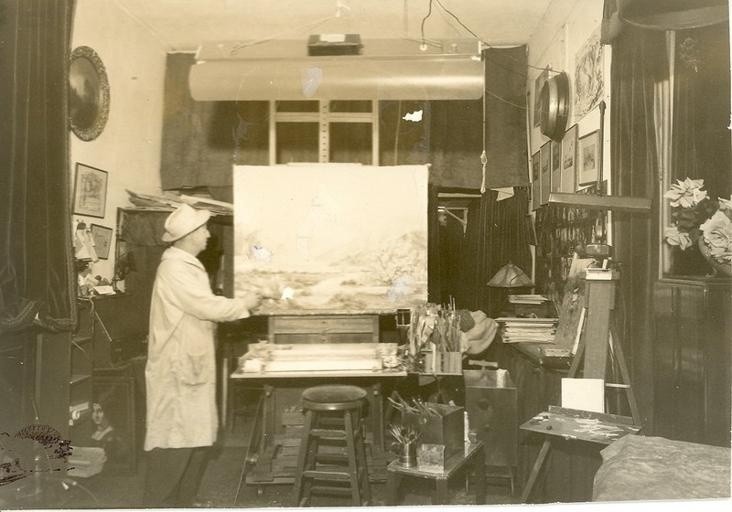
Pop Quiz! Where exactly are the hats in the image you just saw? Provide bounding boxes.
[161,202,211,242]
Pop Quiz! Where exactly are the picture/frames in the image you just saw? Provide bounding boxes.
[90,222,114,260]
[71,161,110,220]
[69,44,113,145]
[530,123,601,213]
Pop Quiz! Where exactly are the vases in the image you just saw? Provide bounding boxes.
[698,233,732,278]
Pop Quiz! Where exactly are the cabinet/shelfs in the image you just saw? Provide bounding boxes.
[78,295,148,474]
[649,274,731,448]
[483,332,583,504]
[34,297,97,480]
[266,313,383,446]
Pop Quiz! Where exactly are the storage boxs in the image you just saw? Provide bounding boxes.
[399,398,467,460]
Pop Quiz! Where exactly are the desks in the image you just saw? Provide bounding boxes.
[407,370,462,403]
[383,440,486,504]
[228,342,409,499]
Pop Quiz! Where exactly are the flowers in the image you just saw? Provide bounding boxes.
[662,177,731,266]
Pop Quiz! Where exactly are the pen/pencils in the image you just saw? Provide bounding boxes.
[388,424,423,447]
[258,294,283,302]
[437,296,461,351]
[386,392,442,418]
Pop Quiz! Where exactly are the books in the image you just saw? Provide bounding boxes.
[70,398,91,413]
[68,445,107,479]
[495,313,558,343]
[126,185,234,215]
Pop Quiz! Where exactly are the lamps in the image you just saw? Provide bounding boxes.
[485,259,536,295]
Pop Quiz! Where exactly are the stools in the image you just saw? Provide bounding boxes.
[298,383,373,506]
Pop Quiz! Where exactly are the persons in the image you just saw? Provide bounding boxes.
[91,398,125,461]
[139,202,265,507]
